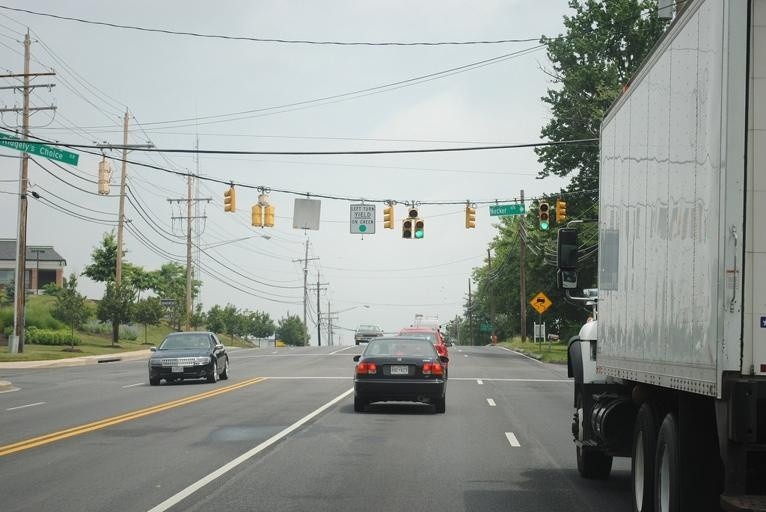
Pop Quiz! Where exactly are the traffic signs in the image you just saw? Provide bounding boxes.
[490,202,525,217]
[480,323,494,331]
[0,132,78,167]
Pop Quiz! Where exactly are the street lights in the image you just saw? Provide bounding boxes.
[185,233,272,329]
[327,304,371,345]
[31,249,45,296]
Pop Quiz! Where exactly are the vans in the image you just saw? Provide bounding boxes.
[410,318,442,334]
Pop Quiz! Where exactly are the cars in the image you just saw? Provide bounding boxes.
[397,327,448,360]
[355,323,383,345]
[351,337,448,413]
[146,331,229,383]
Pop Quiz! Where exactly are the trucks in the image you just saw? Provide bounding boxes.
[557,0,765,510]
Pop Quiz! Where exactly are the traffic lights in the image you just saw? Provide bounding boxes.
[383,208,393,228]
[540,203,551,230]
[402,220,411,237]
[556,201,567,224]
[223,187,235,214]
[416,221,424,237]
[410,208,418,218]
[466,207,475,229]
